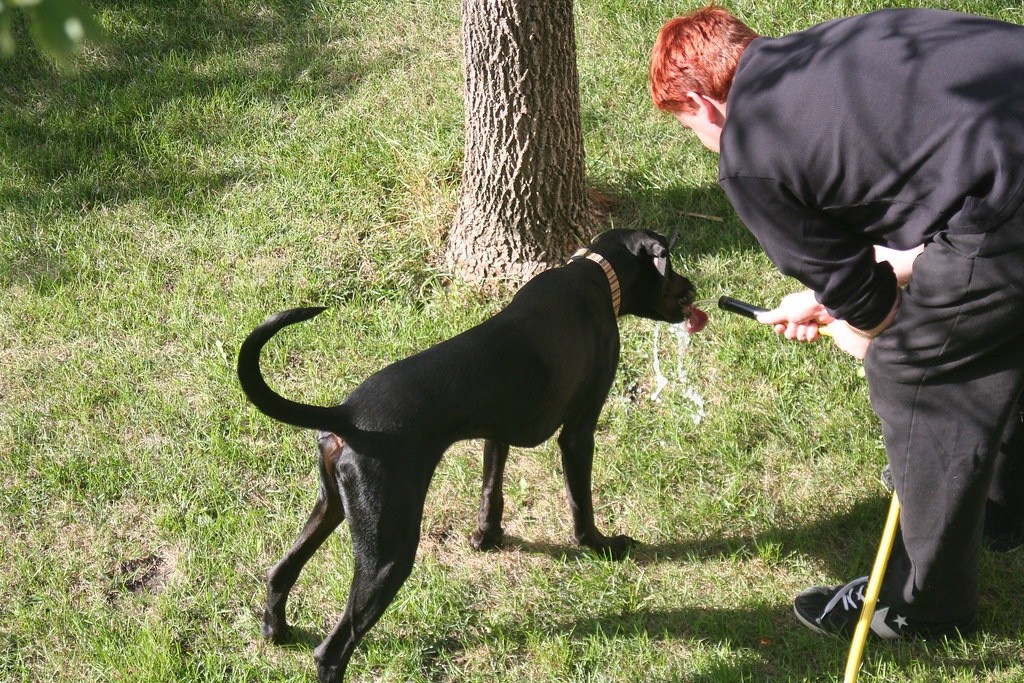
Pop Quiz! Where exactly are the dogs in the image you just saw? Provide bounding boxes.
[236,224,709,683]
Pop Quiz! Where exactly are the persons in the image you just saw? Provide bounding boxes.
[648,3,1024,645]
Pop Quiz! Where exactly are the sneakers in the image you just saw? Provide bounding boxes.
[793,576,977,645]
[880,463,1024,554]
